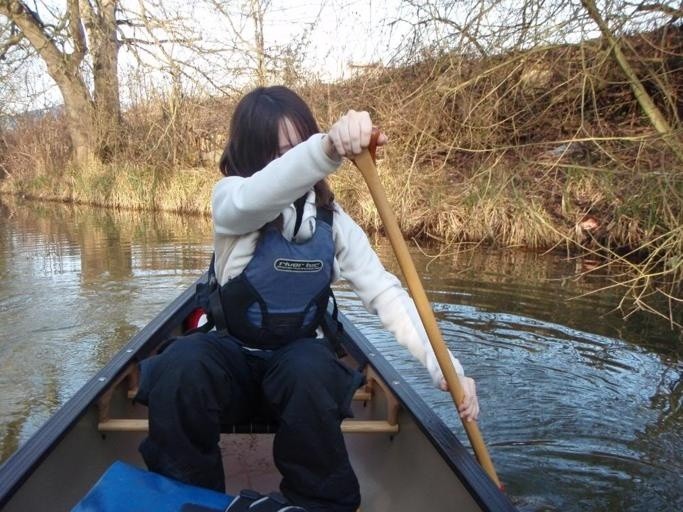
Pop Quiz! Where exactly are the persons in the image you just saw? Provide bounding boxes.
[138,85,480,512]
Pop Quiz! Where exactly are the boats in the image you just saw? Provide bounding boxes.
[0,270,519,512]
